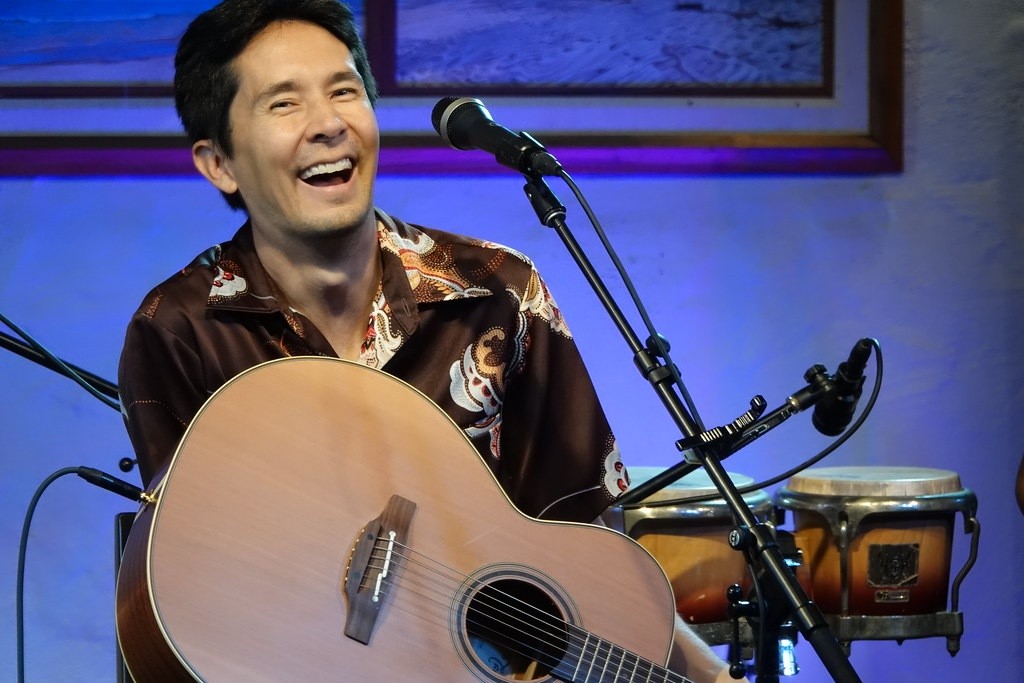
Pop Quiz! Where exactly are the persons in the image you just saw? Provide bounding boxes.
[117,1,761,683]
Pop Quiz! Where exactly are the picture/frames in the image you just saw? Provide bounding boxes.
[0,0,917,183]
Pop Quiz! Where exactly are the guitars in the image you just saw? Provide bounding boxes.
[116,353,700,683]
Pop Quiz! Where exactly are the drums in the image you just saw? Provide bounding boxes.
[615,460,774,648]
[786,466,972,641]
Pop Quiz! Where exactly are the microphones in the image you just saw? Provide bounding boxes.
[811,338,872,438]
[432,97,562,177]
